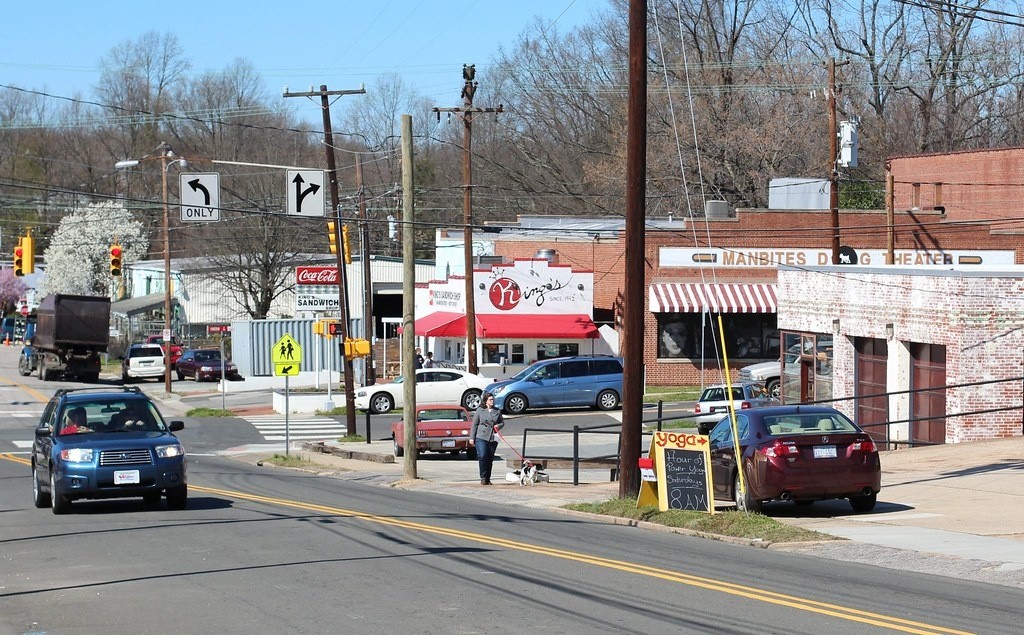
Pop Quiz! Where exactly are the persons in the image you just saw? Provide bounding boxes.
[470,393,505,484]
[415,348,424,381]
[422,351,434,381]
[117,406,146,430]
[73,407,87,426]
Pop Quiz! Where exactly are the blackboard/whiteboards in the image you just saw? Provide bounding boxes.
[637,431,714,515]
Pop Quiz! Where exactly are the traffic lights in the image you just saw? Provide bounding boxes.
[110,245,122,276]
[328,323,343,335]
[338,342,351,356]
[14,247,25,277]
[327,223,350,263]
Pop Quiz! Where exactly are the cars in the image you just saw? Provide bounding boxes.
[391,404,478,460]
[708,406,881,515]
[176,349,237,382]
[354,369,500,414]
[694,384,781,435]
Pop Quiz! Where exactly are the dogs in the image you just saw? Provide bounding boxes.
[520,463,542,486]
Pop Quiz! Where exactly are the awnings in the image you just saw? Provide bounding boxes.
[649,282,778,313]
[397,311,600,339]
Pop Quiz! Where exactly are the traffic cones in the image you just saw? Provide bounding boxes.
[4,332,10,346]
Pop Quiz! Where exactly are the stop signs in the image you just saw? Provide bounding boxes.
[20,306,30,316]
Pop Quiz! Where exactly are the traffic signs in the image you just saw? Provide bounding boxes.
[179,174,220,222]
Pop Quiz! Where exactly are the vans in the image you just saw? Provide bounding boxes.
[480,353,624,415]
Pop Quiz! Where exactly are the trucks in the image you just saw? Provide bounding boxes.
[19,294,111,382]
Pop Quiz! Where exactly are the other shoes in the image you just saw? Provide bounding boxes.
[481,477,492,485]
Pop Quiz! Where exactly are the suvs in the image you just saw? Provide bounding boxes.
[0,318,24,344]
[148,336,185,368]
[737,340,833,397]
[118,344,166,383]
[31,387,187,514]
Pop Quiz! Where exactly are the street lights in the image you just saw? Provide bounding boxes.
[114,156,173,393]
